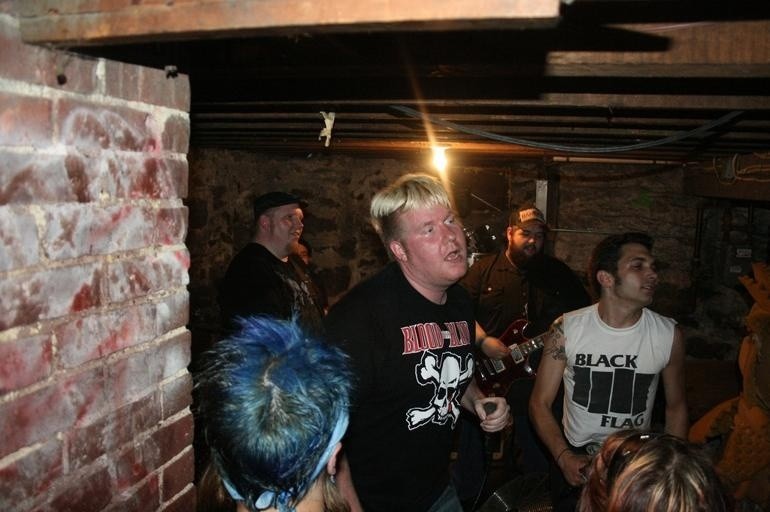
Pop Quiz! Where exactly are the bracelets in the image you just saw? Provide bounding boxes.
[554,445,571,466]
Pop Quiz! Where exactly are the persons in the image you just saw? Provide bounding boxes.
[296,238,313,265]
[527,231,691,510]
[456,204,596,511]
[321,172,512,512]
[217,191,326,339]
[192,315,361,512]
[578,427,729,511]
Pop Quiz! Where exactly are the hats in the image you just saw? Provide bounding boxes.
[253,191,310,216]
[508,206,551,234]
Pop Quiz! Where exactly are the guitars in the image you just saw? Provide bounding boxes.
[475,319,547,394]
